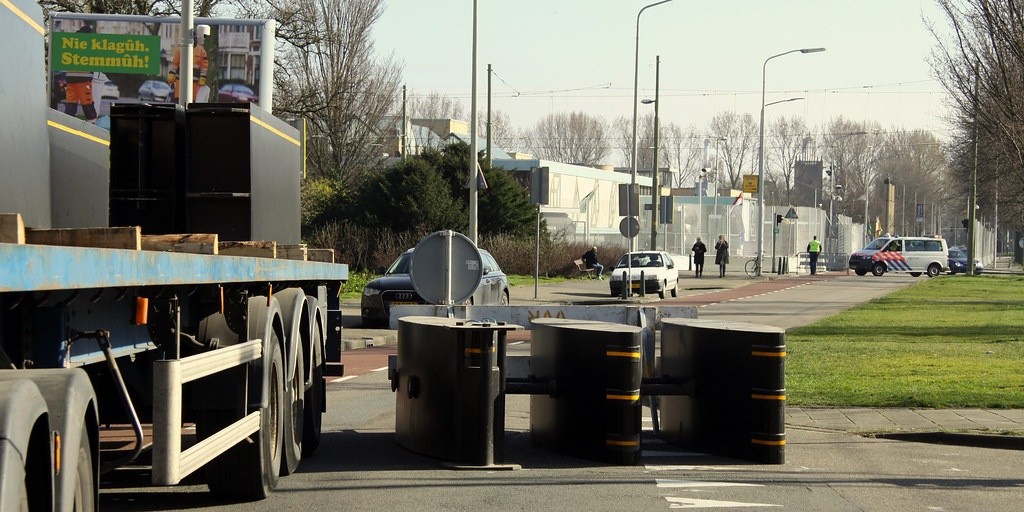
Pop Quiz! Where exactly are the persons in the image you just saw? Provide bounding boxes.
[715,235,729,278]
[692,236,707,278]
[582,246,604,280]
[807,236,822,275]
[890,242,898,251]
[65,26,97,124]
[168,30,209,103]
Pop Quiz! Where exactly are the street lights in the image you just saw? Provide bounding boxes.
[640,55,661,250]
[757,96,804,277]
[757,47,827,279]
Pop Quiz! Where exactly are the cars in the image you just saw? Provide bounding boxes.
[609,250,679,300]
[360,247,512,327]
[101,85,120,100]
[218,83,259,103]
[138,80,172,102]
[948,249,983,276]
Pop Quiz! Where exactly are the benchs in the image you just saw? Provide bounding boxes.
[574,259,596,281]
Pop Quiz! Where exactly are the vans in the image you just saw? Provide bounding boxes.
[849,235,949,278]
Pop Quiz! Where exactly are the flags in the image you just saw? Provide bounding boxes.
[732,194,742,205]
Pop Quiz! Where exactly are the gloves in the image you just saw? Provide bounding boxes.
[166,71,176,86]
[198,77,206,86]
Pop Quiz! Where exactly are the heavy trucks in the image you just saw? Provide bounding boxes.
[0,230,351,512]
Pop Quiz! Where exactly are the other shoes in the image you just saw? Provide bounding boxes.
[596,276,602,281]
[723,275,725,276]
[719,275,722,277]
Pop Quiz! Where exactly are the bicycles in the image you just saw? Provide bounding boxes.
[744,251,766,278]
